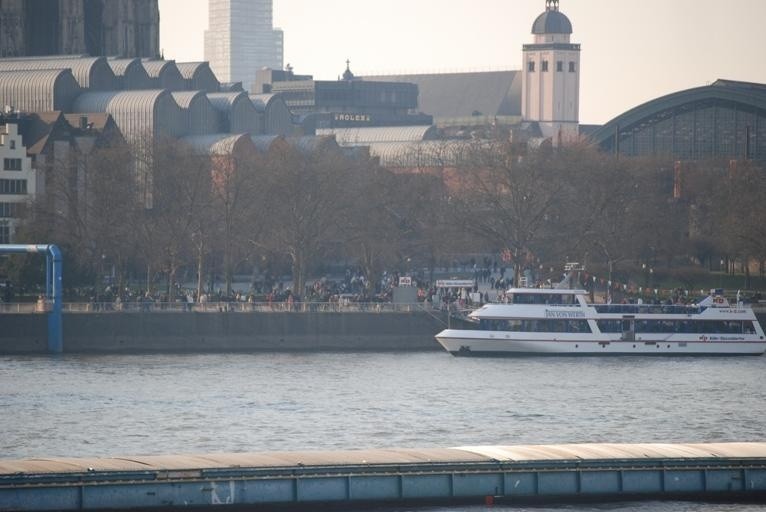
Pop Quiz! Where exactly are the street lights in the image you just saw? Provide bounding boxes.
[642,262,654,286]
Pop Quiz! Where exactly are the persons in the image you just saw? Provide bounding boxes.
[293,294,391,313]
[88,293,169,311]
[418,264,514,304]
[180,282,290,312]
[607,295,698,304]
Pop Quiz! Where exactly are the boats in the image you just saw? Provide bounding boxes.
[435,285,766,357]
